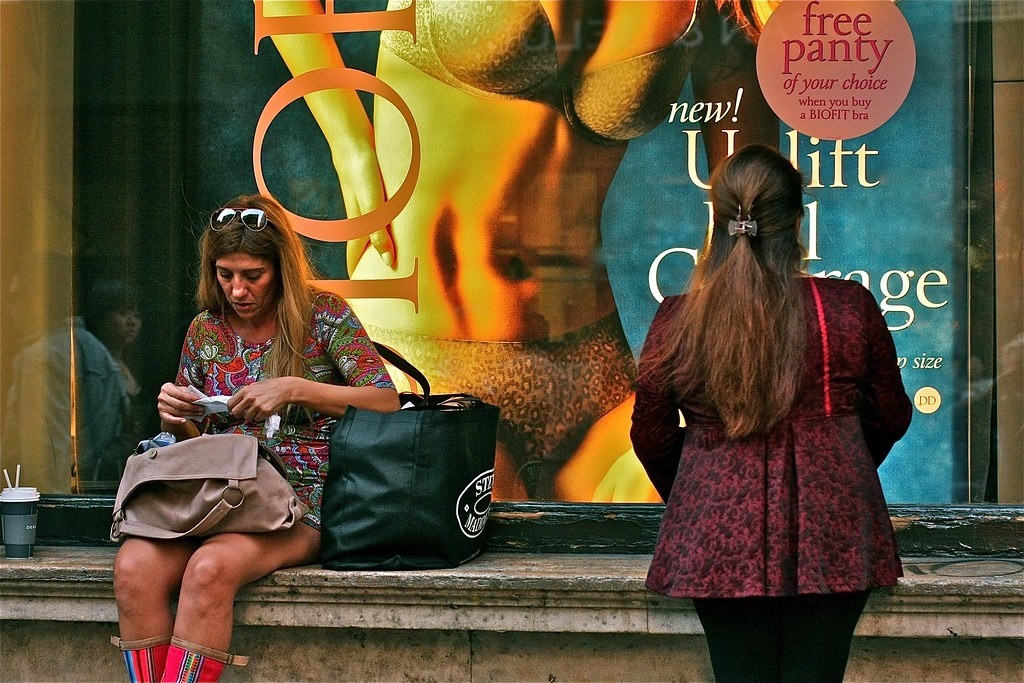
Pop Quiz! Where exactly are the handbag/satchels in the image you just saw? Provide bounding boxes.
[109,434,310,542]
[321,340,501,571]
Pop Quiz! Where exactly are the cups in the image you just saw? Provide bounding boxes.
[0,488,40,560]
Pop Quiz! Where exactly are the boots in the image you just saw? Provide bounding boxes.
[161,636,250,683]
[111,634,174,683]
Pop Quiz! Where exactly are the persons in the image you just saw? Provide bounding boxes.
[629,144,913,683]
[263,0,783,506]
[8,254,142,498]
[112,197,401,683]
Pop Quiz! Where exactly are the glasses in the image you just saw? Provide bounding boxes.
[210,208,273,231]
